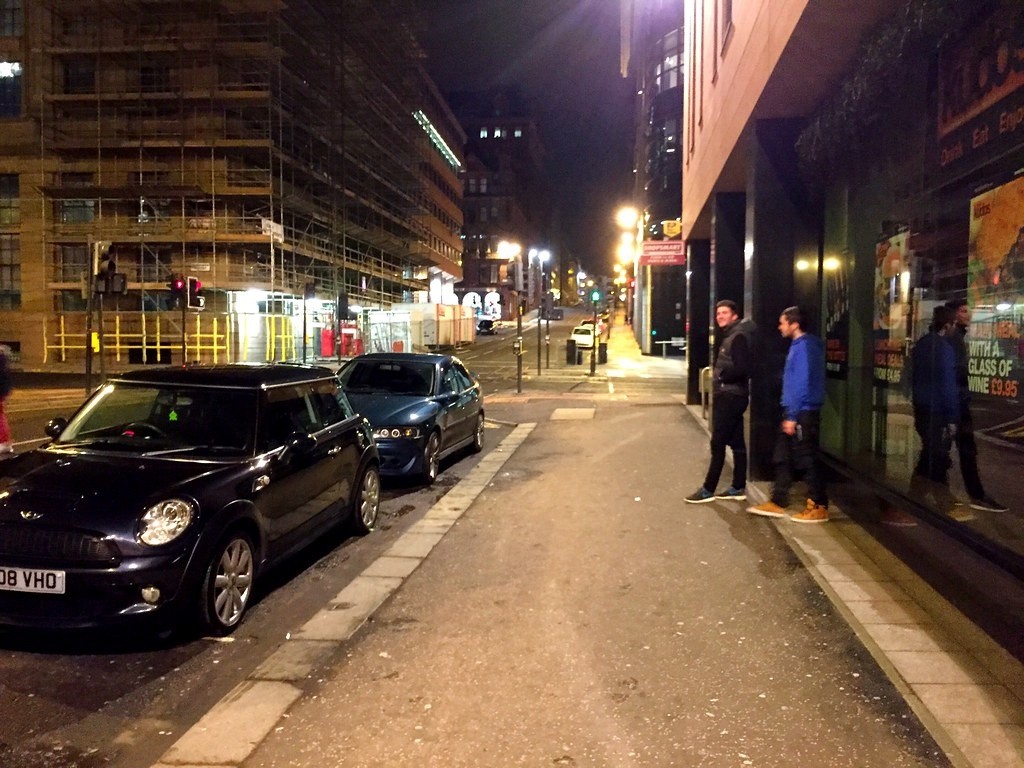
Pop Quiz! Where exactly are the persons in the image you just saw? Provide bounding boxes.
[0,349,15,453]
[684,300,758,503]
[745,307,829,522]
[909,301,1009,521]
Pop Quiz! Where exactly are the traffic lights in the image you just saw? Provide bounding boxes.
[590,290,601,301]
[94,241,118,276]
[171,273,185,296]
[187,276,205,309]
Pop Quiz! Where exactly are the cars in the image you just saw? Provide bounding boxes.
[548,309,563,320]
[580,320,602,336]
[569,327,594,347]
[0,363,382,637]
[476,320,498,336]
[336,352,486,485]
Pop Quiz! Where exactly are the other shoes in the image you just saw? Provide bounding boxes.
[0,444,12,454]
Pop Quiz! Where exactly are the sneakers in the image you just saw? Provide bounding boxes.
[683,487,716,504]
[968,495,1009,513]
[880,507,918,526]
[715,487,746,500]
[946,503,979,522]
[746,500,785,518]
[930,493,965,506]
[790,498,830,524]
